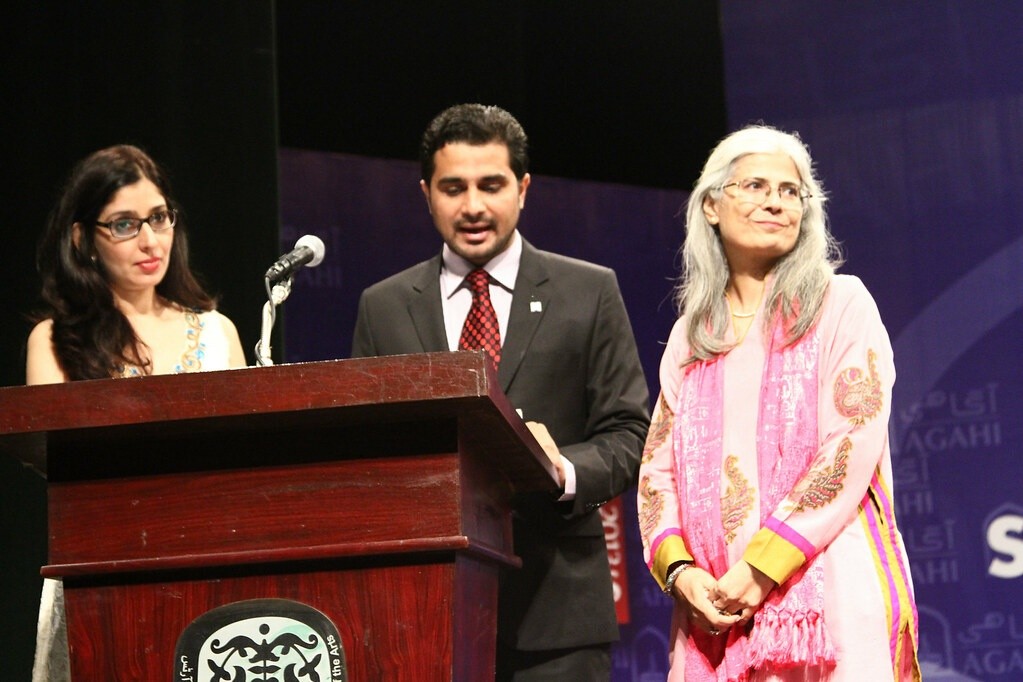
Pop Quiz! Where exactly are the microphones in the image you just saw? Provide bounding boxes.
[265,235,326,282]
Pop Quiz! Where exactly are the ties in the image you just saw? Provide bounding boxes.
[458,271,501,370]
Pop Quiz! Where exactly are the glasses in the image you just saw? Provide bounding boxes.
[719,179,812,212]
[94,209,178,239]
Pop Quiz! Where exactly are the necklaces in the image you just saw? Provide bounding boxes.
[731,313,755,319]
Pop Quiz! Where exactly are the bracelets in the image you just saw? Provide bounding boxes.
[664,563,689,594]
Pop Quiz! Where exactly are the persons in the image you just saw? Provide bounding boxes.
[350,102,652,682]
[27,143,248,682]
[637,125,919,682]
